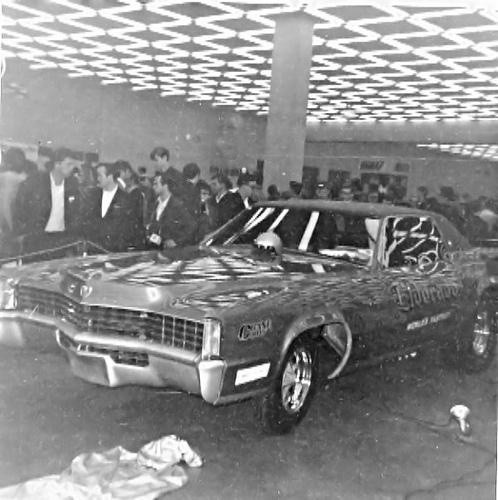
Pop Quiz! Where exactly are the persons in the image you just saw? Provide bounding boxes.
[1,139,498,266]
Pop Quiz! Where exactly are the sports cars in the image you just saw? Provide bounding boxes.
[0,195,497,441]
